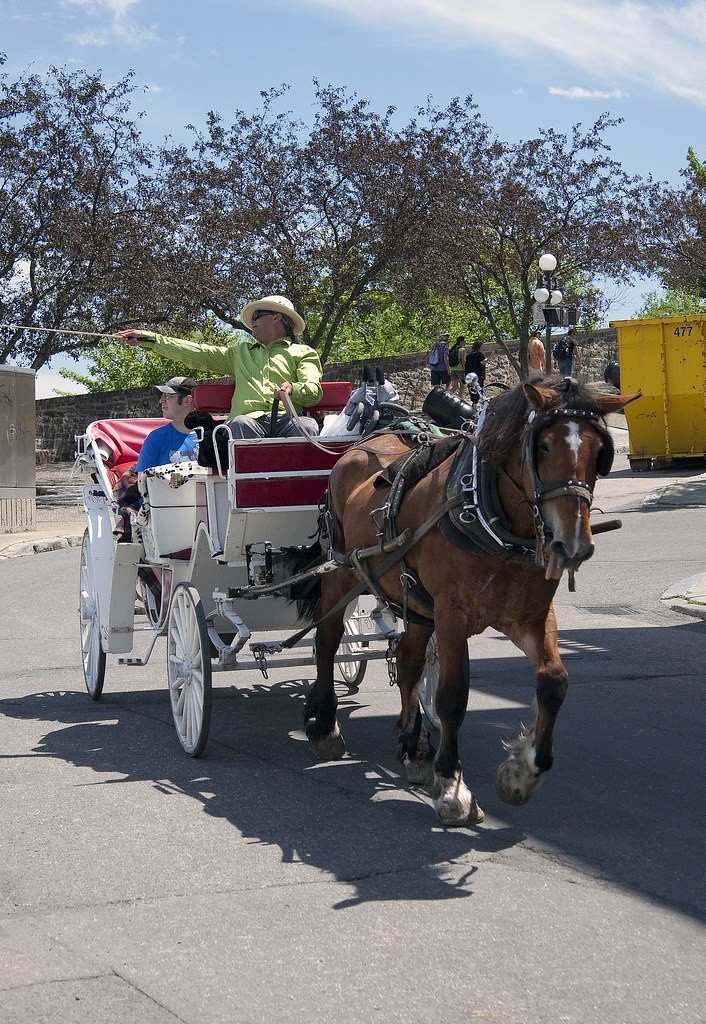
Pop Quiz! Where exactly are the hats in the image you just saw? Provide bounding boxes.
[152,377,197,396]
[439,331,450,336]
[239,295,305,334]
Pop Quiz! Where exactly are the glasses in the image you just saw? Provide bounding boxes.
[159,393,181,399]
[252,312,275,321]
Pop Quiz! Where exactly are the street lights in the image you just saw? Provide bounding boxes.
[532,253,563,375]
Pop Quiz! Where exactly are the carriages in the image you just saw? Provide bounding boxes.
[72,371,646,832]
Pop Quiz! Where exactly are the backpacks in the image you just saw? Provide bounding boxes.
[428,343,440,366]
[553,337,570,361]
[448,344,461,367]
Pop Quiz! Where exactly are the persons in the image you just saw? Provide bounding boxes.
[114,293,322,440]
[465,342,496,408]
[527,332,546,379]
[428,330,452,390]
[449,335,467,400]
[557,329,584,378]
[130,376,209,518]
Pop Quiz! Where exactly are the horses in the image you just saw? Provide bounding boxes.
[271,370,643,826]
